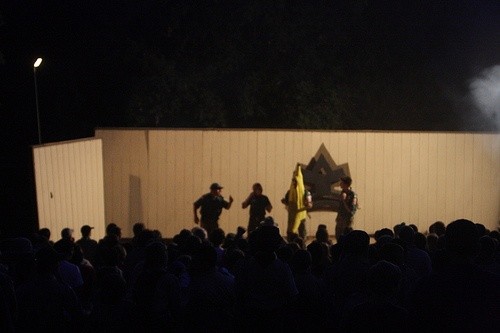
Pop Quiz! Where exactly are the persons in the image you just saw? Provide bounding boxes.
[191,183,232,232]
[241,178,313,245]
[0,219,500,333]
[334,176,357,244]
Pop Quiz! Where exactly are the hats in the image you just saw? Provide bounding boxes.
[210,183,223,189]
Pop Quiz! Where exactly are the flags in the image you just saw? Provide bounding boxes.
[293,168,307,235]
